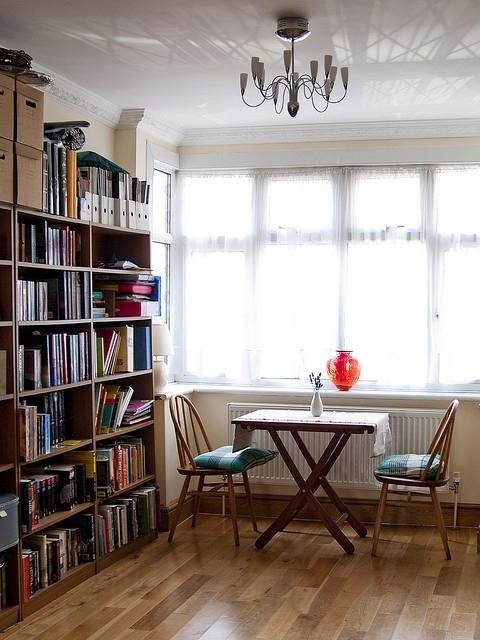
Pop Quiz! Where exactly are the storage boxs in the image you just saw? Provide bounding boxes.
[15,79,45,152]
[0,136,15,205]
[15,140,43,212]
[0,72,15,141]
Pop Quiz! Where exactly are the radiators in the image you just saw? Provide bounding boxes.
[226,402,451,496]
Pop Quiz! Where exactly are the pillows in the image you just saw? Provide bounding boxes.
[373,453,446,480]
[188,444,280,473]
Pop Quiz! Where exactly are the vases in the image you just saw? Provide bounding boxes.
[324,350,363,392]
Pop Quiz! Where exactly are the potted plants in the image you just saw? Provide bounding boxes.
[309,370,324,417]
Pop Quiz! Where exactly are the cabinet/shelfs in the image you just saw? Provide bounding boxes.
[0,202,23,634]
[15,203,160,622]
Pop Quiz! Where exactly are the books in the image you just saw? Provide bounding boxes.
[1,350,6,395]
[96,437,146,498]
[17,221,96,535]
[21,513,95,602]
[92,260,162,435]
[97,484,156,556]
[44,142,150,219]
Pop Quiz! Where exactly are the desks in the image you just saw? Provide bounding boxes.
[229,409,391,556]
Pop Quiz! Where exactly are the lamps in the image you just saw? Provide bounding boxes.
[151,320,177,398]
[239,1,350,120]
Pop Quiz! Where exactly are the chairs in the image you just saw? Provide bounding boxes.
[370,397,460,561]
[167,392,258,547]
